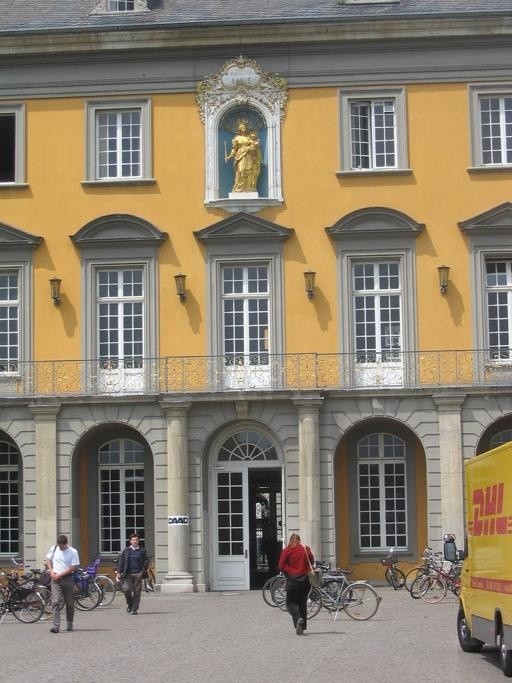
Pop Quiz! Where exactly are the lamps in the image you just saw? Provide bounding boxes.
[49,277,62,305]
[437,265,450,293]
[173,274,186,301]
[303,270,316,299]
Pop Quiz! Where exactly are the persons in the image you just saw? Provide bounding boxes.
[236,129,260,163]
[46,534,81,632]
[224,120,260,191]
[278,532,315,635]
[117,533,150,615]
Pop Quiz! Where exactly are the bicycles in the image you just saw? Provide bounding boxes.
[381,545,406,589]
[404,545,464,602]
[0,556,117,623]
[262,558,383,621]
[111,552,155,592]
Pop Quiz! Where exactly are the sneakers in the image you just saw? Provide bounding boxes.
[127,606,132,612]
[66,622,73,630]
[132,609,138,615]
[296,618,304,635]
[50,626,60,633]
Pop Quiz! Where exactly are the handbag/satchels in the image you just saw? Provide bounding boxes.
[307,567,322,588]
[39,568,51,592]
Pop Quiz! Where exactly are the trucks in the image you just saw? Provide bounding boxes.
[455,438,512,678]
